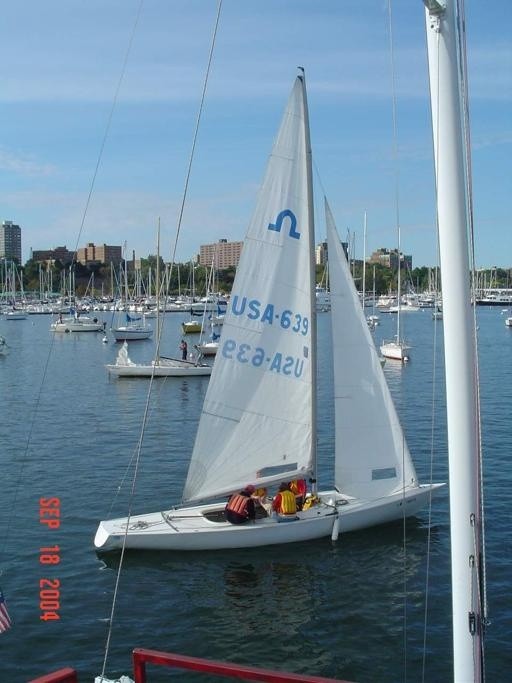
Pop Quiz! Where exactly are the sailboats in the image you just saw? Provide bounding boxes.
[179,258,203,332]
[378,226,413,362]
[0,238,229,325]
[315,209,511,333]
[84,64,441,552]
[192,261,221,355]
[104,216,213,376]
[50,265,108,333]
[109,240,154,340]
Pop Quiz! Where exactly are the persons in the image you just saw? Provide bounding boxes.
[288,479,307,512]
[59,312,62,324]
[251,471,267,519]
[224,484,255,524]
[271,482,296,519]
[178,339,187,360]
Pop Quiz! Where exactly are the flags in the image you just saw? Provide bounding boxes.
[0,589,12,634]
